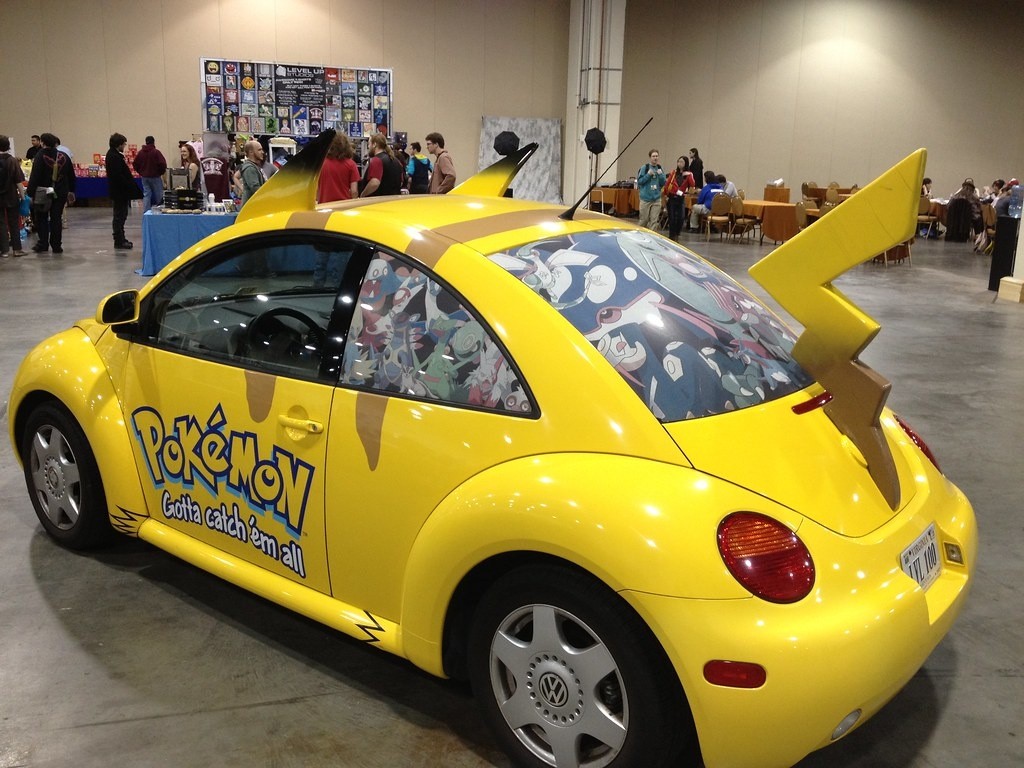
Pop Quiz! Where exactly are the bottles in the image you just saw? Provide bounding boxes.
[634,178,637,189]
[1008,185,1024,218]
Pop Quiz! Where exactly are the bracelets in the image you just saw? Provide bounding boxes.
[21,192,25,194]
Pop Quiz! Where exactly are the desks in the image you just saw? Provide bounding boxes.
[590,186,632,214]
[76,171,142,210]
[729,199,799,245]
[929,198,986,234]
[805,208,820,218]
[806,186,852,207]
[141,206,238,276]
[629,188,697,229]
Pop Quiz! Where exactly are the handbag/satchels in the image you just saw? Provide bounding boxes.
[33,187,55,212]
[660,183,672,196]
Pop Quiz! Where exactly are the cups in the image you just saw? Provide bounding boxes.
[209,193,214,204]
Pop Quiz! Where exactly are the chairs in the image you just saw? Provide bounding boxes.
[701,181,996,267]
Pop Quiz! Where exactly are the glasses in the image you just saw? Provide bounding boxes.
[426,142,434,145]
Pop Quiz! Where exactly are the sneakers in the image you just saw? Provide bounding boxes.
[13,250,29,256]
[113,240,132,249]
[1,253,9,257]
[50,240,63,253]
[32,240,49,253]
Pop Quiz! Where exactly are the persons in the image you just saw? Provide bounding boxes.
[637,149,666,231]
[687,171,738,233]
[233,141,279,207]
[426,132,456,194]
[919,178,942,237]
[105,133,133,249]
[0,133,76,258]
[663,156,695,243]
[316,131,361,204]
[359,133,434,198]
[947,176,1019,236]
[181,144,208,199]
[689,148,703,190]
[132,136,167,213]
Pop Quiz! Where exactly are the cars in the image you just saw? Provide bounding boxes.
[7,127,981,768]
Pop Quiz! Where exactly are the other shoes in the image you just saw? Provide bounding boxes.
[687,229,699,233]
